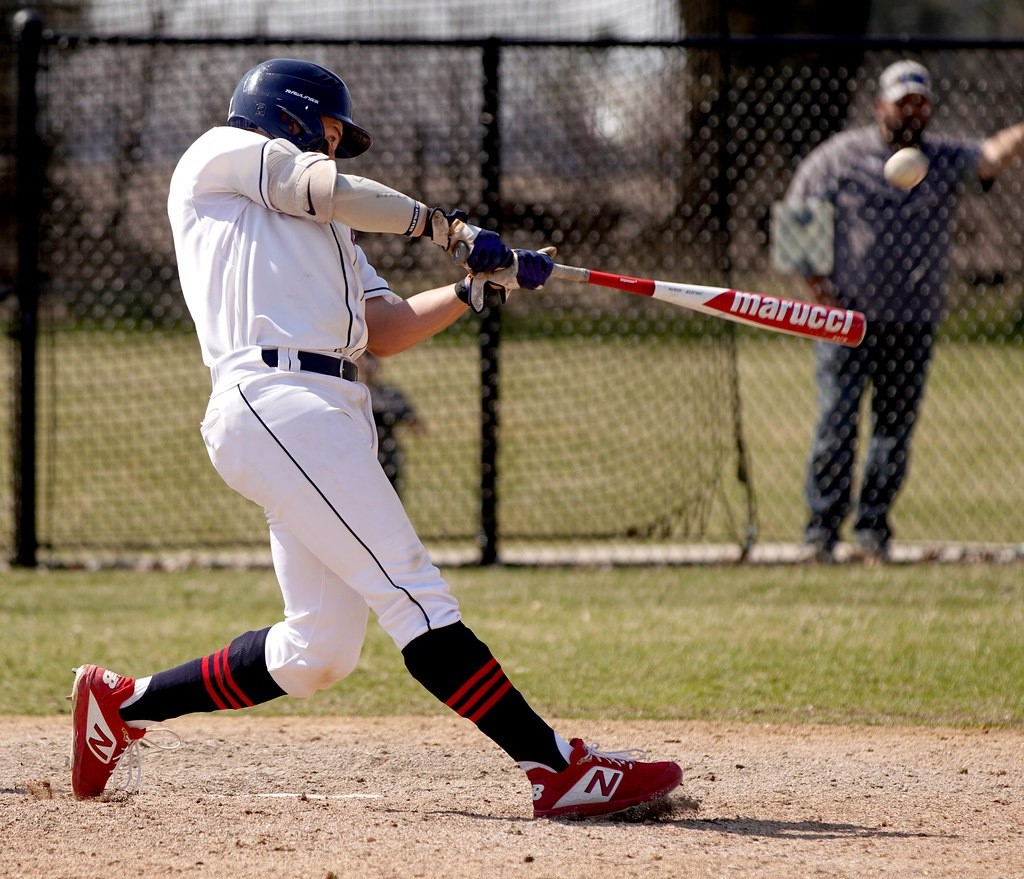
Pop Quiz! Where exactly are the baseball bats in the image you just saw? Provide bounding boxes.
[452,242,867,350]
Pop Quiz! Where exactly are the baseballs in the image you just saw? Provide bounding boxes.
[881,146,931,191]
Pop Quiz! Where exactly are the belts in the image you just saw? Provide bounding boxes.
[262,349,358,382]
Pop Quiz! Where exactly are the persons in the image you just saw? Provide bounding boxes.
[352,316,438,527]
[771,55,1024,566]
[71,58,681,815]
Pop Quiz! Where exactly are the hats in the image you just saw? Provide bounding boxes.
[880,60,932,103]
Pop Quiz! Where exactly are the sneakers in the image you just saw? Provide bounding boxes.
[525,738,684,819]
[65,664,181,802]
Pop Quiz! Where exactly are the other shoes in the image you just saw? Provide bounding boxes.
[805,540,889,563]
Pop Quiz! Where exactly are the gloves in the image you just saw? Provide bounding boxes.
[429,208,514,273]
[464,246,557,314]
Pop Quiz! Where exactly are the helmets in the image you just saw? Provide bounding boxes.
[228,58,373,159]
